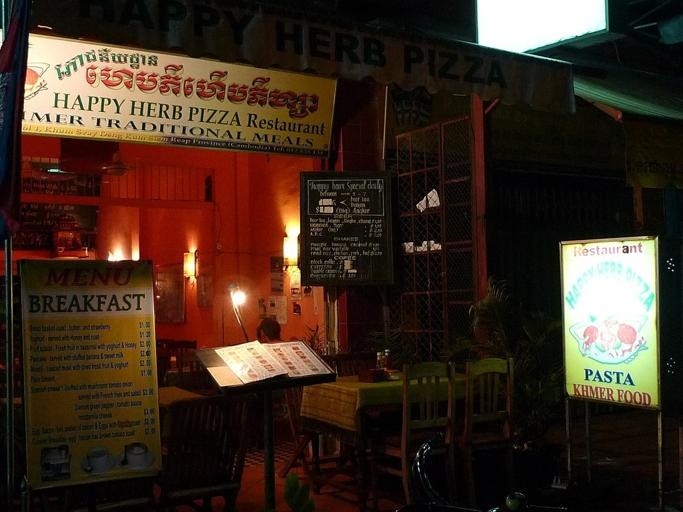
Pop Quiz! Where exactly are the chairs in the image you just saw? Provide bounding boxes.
[360,358,453,509]
[440,352,514,507]
[164,387,253,510]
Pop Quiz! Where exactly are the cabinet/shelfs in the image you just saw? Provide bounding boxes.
[0,133,105,276]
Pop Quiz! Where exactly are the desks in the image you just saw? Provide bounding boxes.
[278,371,479,504]
[156,384,202,406]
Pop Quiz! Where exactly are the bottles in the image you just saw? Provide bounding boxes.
[375,349,393,369]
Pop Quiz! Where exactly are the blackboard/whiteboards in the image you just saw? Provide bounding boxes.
[300,170,395,288]
[12,156,102,251]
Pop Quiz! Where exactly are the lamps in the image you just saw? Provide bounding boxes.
[227,285,250,343]
[100,142,128,177]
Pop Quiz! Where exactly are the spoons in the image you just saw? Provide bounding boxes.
[121,446,129,465]
[85,458,93,474]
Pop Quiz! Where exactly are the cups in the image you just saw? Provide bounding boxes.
[89,448,109,471]
[128,443,148,468]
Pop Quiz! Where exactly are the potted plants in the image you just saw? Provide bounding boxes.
[439,281,564,504]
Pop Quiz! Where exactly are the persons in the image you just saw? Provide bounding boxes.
[255,318,285,343]
